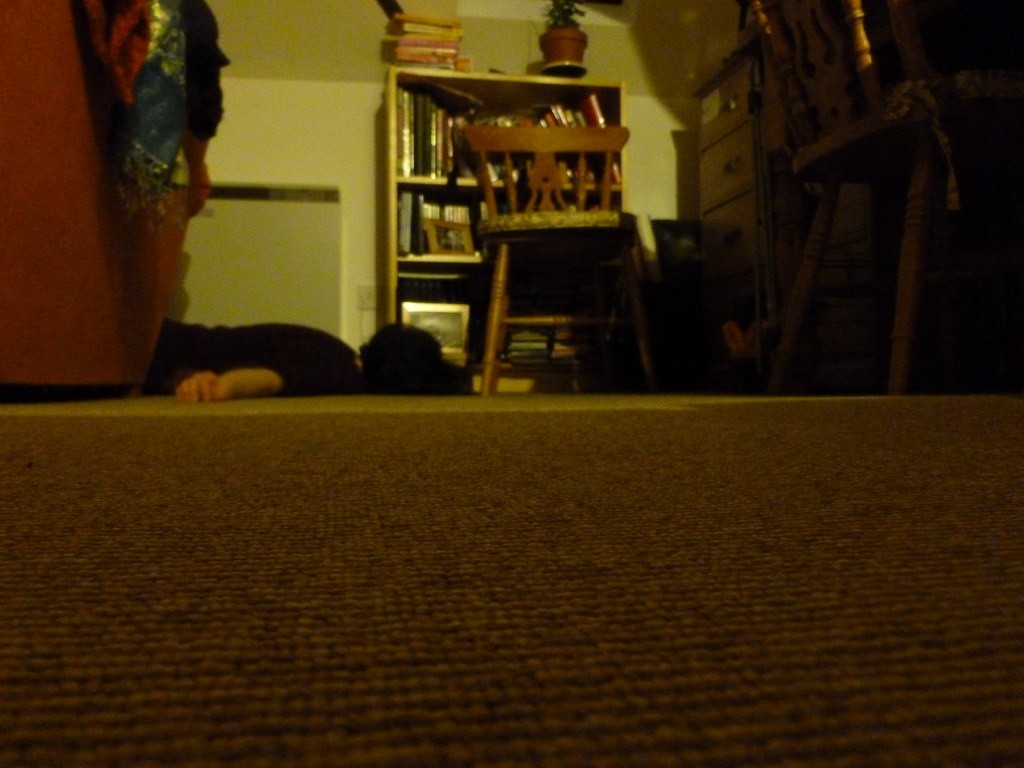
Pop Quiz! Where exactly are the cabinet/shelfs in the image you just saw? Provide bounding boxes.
[382,66,627,392]
[691,21,870,400]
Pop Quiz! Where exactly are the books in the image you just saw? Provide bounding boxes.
[401,91,623,365]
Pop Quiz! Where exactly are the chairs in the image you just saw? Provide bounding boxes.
[462,126,655,400]
[749,0,1024,395]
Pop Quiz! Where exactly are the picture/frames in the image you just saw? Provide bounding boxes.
[402,301,470,359]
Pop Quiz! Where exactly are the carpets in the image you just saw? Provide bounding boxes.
[0,393,1024,768]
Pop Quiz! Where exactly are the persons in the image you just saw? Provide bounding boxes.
[2,317,449,401]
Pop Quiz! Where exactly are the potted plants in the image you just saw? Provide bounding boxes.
[538,0,588,78]
